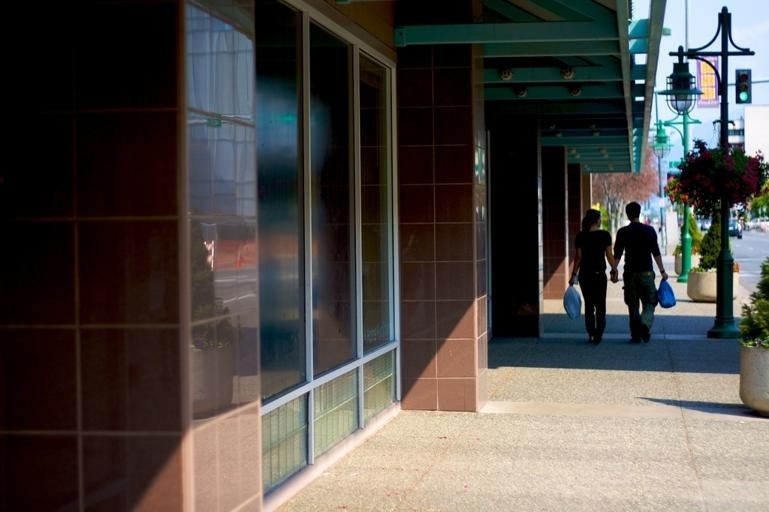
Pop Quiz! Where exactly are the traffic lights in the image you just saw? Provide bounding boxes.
[736,69,751,104]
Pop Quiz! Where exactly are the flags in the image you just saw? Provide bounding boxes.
[695,54,719,109]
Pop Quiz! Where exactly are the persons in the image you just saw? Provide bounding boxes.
[570,208,618,343]
[610,201,668,344]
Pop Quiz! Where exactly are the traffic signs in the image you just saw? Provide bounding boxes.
[206,118,221,127]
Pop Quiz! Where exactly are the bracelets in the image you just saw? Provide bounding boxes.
[661,270,665,274]
[610,271,615,274]
[572,273,577,277]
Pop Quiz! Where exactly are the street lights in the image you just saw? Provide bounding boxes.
[656,4,759,338]
[648,105,703,282]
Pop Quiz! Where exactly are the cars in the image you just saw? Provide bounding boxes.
[645,206,769,239]
[192,207,322,369]
